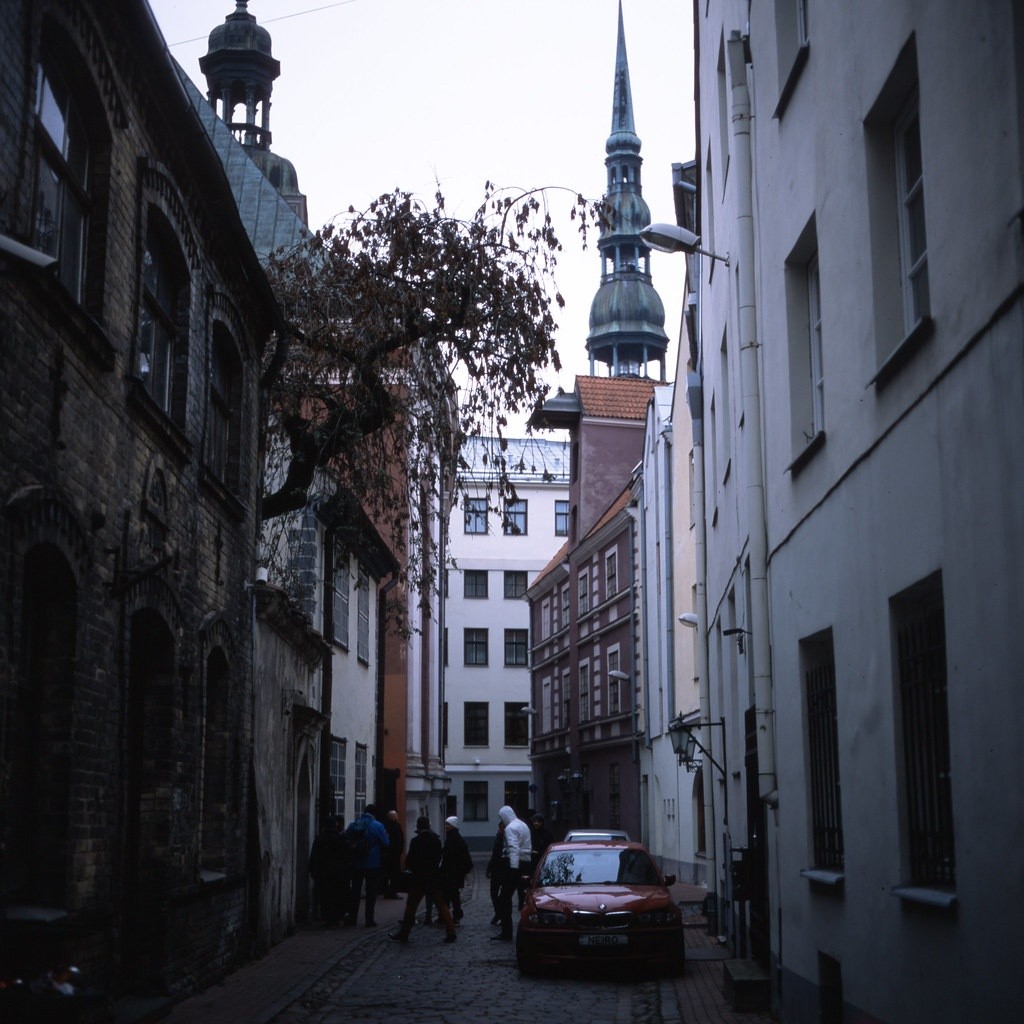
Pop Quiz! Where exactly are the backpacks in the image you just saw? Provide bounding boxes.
[347,819,373,871]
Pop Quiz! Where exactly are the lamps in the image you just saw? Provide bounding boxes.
[679,614,698,626]
[637,222,730,265]
[521,707,537,715]
[676,738,703,774]
[668,711,729,826]
[608,671,629,681]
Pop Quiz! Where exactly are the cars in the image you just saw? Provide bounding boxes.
[516,829,686,978]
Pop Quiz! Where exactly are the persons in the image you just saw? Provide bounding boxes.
[490,822,508,925]
[388,816,457,943]
[520,809,550,877]
[342,804,391,927]
[381,810,404,900]
[309,814,353,930]
[489,806,532,941]
[435,814,473,927]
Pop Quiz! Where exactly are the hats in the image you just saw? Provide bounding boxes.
[445,816,460,830]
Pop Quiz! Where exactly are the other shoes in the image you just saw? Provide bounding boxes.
[490,913,500,925]
[388,932,408,944]
[398,919,405,924]
[454,919,460,923]
[442,935,457,942]
[365,921,377,928]
[384,891,403,899]
[490,933,512,942]
[423,919,433,925]
